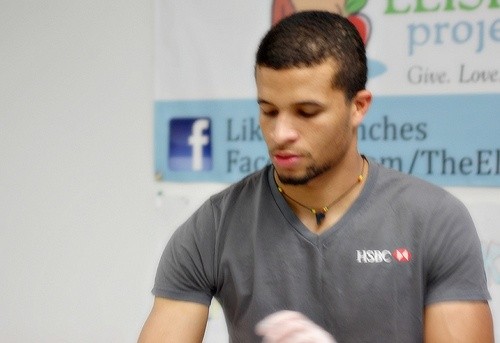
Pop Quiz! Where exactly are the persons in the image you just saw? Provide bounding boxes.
[138,10,495,343]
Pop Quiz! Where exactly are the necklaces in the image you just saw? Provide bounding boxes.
[278,158,364,226]
